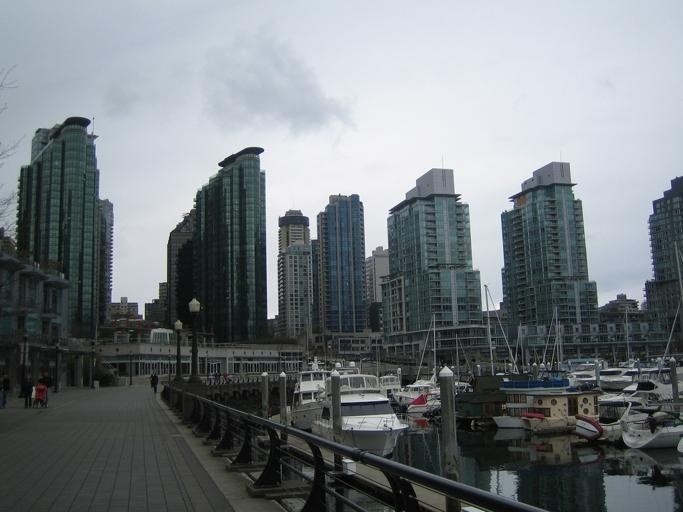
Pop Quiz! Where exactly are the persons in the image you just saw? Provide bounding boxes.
[0,372,48,408]
[151,373,158,393]
[149,371,154,388]
[214,369,221,382]
[86,376,100,394]
[222,369,229,384]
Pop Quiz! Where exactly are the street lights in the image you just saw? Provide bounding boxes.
[129,350,133,386]
[189,297,200,383]
[19,333,28,397]
[174,320,183,382]
[168,354,171,383]
[54,339,60,392]
[91,340,95,387]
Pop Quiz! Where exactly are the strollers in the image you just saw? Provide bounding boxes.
[32,384,48,408]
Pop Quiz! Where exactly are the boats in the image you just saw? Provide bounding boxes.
[311,373,410,459]
[290,285,683,457]
